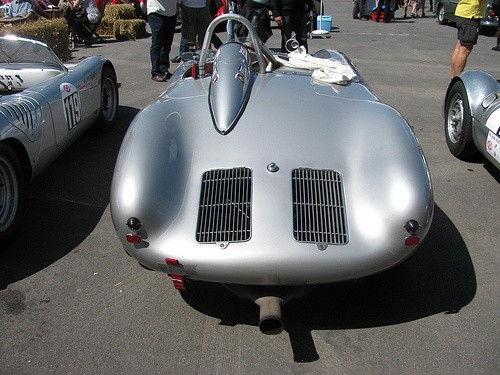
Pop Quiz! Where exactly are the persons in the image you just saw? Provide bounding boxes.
[349,0,435,24]
[450,0,489,81]
[491,0,500,51]
[0,0,325,63]
[146,0,178,82]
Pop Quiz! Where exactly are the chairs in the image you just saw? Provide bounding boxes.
[52,6,105,47]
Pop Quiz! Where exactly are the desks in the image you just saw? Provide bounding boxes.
[0,0,65,23]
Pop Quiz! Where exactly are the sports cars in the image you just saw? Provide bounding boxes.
[111,13,434,337]
[0,36,121,247]
[443,69,500,177]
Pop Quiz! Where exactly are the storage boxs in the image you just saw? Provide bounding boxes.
[317,15,332,31]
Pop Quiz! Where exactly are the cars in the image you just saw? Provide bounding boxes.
[434,0,499,36]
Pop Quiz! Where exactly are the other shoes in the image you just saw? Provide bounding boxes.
[171,55,182,63]
[152,74,166,82]
[162,71,173,79]
[84,36,93,47]
